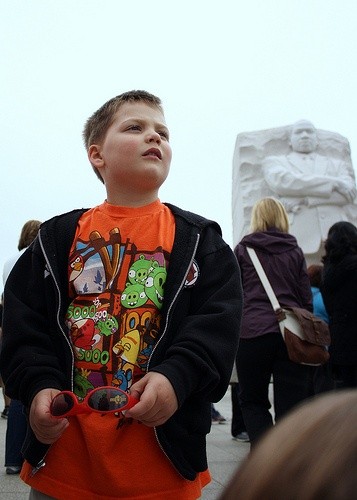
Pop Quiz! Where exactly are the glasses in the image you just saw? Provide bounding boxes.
[49,386,140,424]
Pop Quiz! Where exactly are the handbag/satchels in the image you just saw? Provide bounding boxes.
[277,307,331,367]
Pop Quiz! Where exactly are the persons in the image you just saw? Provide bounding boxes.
[0,89,244,500]
[303,264,329,354]
[0,379,13,419]
[211,403,227,424]
[3,220,43,474]
[218,389,357,500]
[232,196,313,454]
[319,220,357,391]
[229,355,251,442]
[262,119,357,253]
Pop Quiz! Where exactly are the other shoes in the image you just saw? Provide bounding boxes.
[2,409,9,419]
[5,467,22,474]
[212,416,226,424]
[232,432,249,442]
